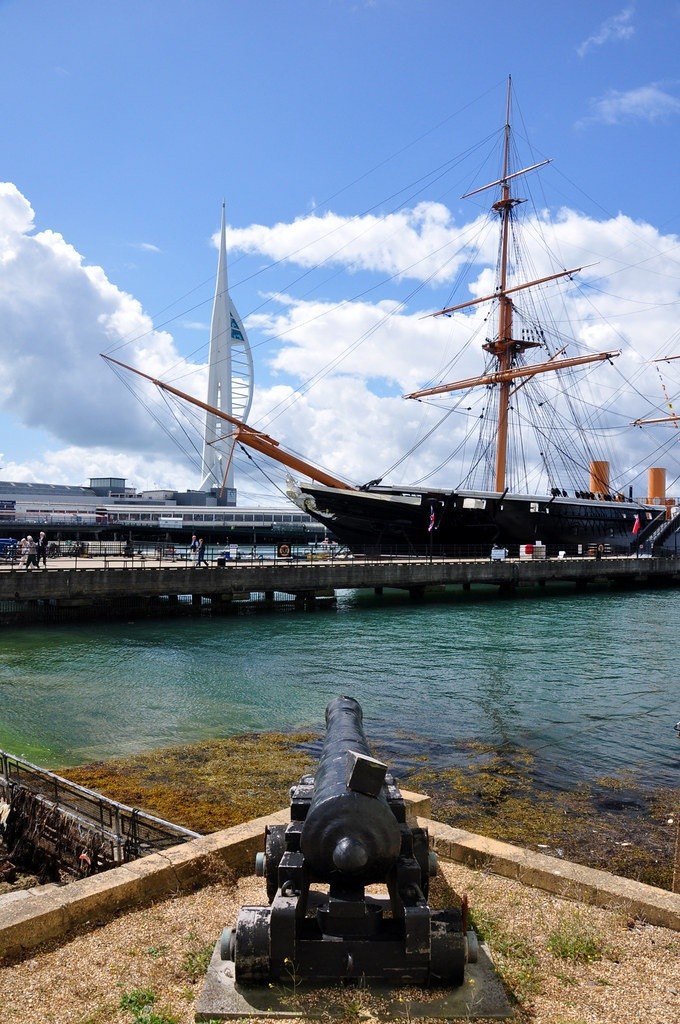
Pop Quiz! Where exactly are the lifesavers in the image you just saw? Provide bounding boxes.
[598,545,603,552]
[278,544,291,557]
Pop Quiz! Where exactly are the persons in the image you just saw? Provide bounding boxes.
[17,536,40,570]
[37,531,48,566]
[190,535,209,566]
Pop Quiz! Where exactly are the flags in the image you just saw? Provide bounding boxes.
[632,514,641,535]
[427,505,435,533]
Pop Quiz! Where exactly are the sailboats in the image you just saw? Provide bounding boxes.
[98,67,680,561]
